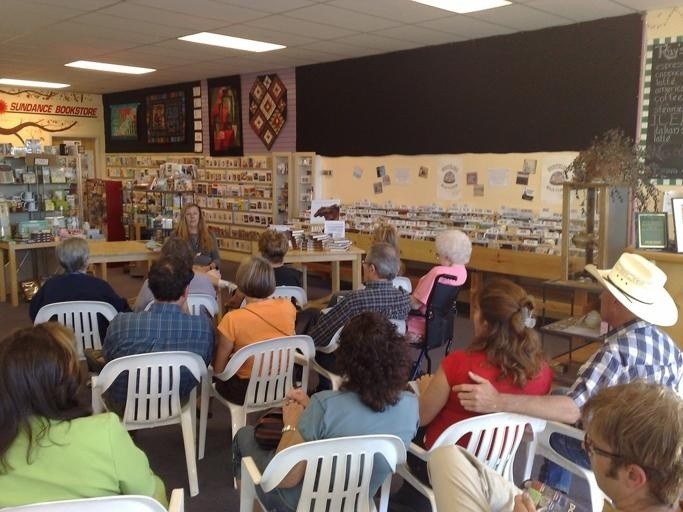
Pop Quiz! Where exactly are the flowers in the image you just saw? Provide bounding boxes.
[560,128,662,211]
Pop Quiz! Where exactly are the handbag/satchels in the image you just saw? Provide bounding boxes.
[253,407,283,450]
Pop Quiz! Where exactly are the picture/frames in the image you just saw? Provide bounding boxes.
[636,212,669,252]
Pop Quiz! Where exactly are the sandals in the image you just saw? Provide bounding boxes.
[84,349,104,373]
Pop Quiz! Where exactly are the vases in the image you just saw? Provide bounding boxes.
[586,160,608,179]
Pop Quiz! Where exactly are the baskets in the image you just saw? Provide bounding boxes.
[31,233,51,242]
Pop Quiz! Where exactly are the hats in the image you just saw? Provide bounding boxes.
[583,252,679,326]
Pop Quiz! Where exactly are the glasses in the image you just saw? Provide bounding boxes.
[584,432,625,462]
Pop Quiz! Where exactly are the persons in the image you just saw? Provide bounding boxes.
[83,238,216,420]
[405,277,553,512]
[452,252,683,494]
[169,204,219,270]
[252,312,420,512]
[195,230,304,418]
[297,225,472,392]
[29,237,132,347]
[428,383,683,512]
[1,324,169,509]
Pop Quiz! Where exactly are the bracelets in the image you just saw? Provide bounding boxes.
[282,425,296,432]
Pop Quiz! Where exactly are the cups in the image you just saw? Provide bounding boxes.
[20,191,34,201]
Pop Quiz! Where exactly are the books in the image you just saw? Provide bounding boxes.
[270,224,352,252]
